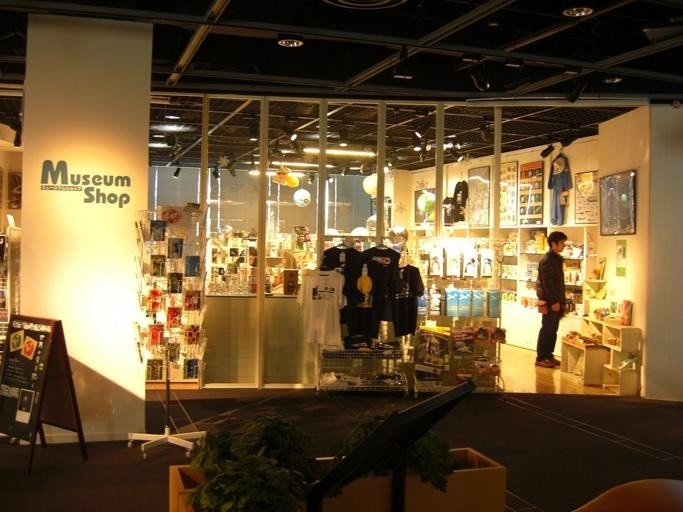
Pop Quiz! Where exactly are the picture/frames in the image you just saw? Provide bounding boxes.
[370,196,391,228]
[415,160,636,235]
[8,172,22,210]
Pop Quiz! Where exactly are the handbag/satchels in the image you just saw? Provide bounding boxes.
[537,300,548,316]
[558,190,571,206]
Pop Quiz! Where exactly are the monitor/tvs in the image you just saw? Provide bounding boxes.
[598,169,637,237]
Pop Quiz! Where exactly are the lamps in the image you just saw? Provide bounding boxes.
[468,54,490,91]
[568,70,586,104]
[275,32,305,47]
[362,163,377,201]
[294,176,311,208]
[541,142,555,157]
[14,132,22,146]
[392,43,411,81]
[562,0,594,18]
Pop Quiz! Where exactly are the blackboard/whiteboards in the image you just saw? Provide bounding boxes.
[0,315,56,443]
[599,169,636,235]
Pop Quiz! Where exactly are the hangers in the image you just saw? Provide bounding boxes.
[375,236,388,250]
[314,255,334,272]
[335,235,351,249]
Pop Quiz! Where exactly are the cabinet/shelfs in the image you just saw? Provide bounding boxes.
[409,227,642,395]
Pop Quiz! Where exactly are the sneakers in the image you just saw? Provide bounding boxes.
[535,356,561,368]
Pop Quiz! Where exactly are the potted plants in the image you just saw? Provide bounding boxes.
[168,419,311,512]
[404,431,507,512]
[312,406,401,512]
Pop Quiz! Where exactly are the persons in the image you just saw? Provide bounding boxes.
[233,246,256,274]
[534,232,570,369]
